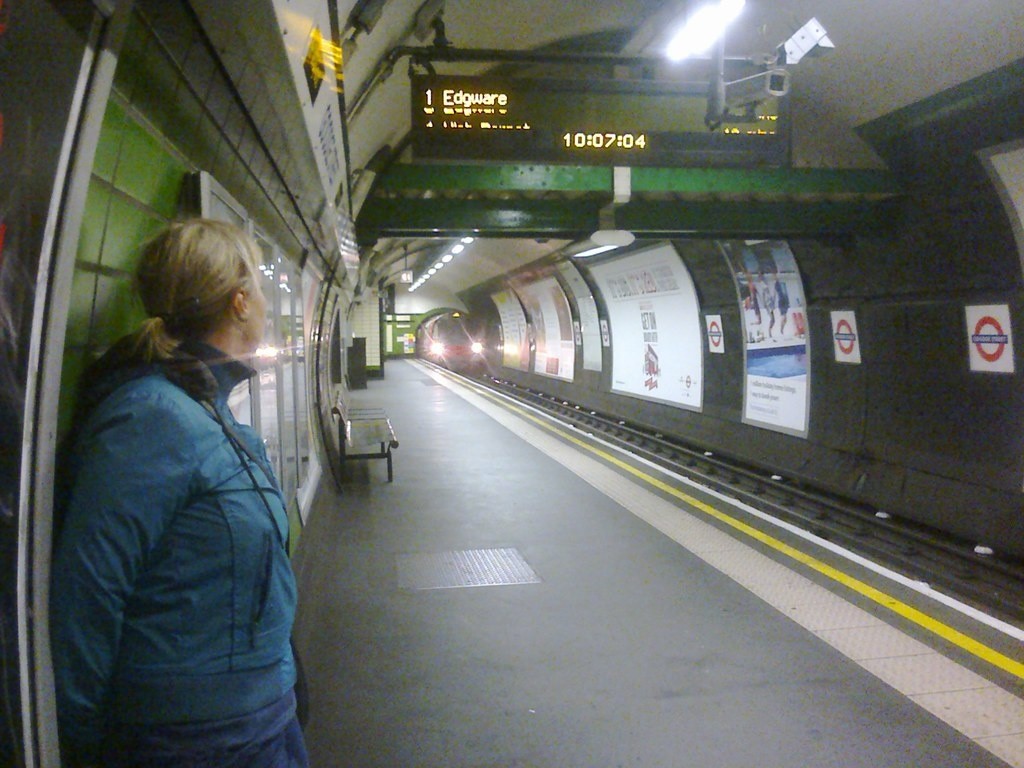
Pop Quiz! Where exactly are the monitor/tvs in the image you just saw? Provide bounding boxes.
[195,170,249,236]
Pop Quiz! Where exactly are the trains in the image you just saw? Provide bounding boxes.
[417,311,485,369]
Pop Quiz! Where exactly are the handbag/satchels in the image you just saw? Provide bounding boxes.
[290,637,308,732]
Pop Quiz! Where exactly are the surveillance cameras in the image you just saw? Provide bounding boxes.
[725,70,790,108]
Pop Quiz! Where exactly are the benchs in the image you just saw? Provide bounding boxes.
[331,390,399,483]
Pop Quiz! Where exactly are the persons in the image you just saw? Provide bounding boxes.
[745,268,790,340]
[50,221,311,768]
